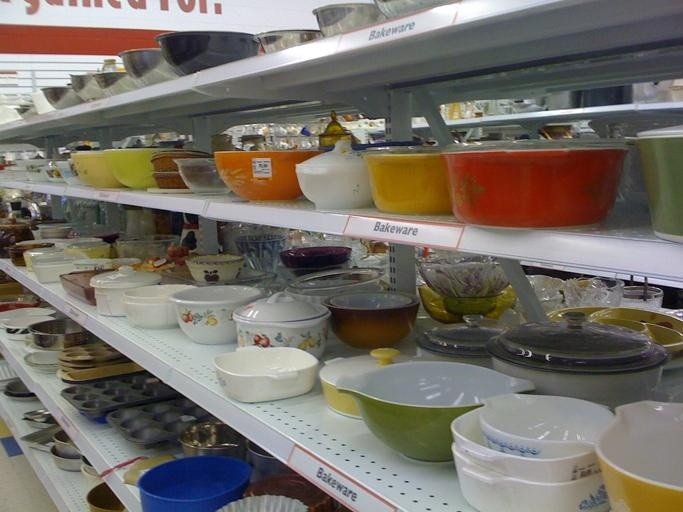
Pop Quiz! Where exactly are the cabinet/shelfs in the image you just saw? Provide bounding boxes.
[0,0,683,512]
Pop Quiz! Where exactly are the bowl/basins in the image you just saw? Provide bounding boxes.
[0,0,683,512]
